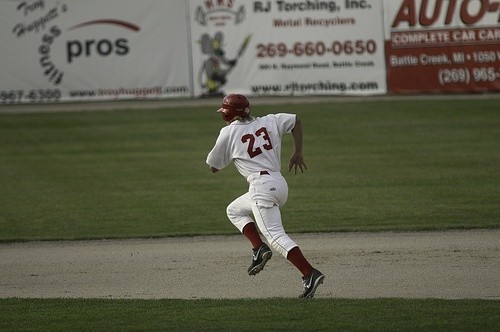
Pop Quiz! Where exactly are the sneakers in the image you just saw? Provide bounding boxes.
[299,269,325,299]
[247,242,272,275]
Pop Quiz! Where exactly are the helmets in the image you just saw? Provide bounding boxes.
[216,93,251,124]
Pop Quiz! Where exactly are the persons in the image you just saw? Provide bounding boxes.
[206,94,326,299]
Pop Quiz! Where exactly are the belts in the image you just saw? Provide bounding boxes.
[260,171,270,176]
